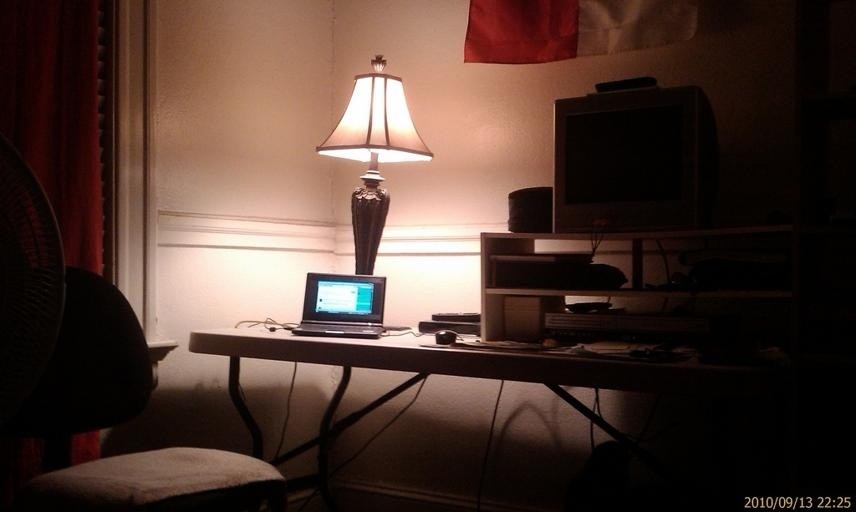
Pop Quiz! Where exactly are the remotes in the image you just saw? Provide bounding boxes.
[595,76,658,92]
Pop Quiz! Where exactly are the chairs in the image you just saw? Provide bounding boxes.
[0,265,288,512]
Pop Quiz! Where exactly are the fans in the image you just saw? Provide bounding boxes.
[0,134,67,395]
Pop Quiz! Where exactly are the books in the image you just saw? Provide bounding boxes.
[570,337,658,359]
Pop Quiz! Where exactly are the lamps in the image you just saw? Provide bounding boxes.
[315,54,434,275]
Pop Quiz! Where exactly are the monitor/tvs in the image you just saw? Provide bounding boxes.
[551,84,722,233]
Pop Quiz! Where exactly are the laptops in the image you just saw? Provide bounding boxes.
[291,271,387,338]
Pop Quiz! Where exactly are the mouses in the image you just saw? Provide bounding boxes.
[435,329,457,344]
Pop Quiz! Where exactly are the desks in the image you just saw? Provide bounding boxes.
[189,224,801,512]
